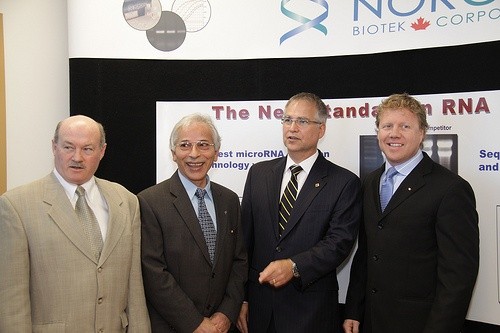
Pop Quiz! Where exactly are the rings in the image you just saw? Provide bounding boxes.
[273,279,276,283]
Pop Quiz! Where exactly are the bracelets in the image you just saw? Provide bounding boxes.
[290,259,300,277]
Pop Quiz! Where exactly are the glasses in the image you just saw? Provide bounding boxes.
[174,141,216,153]
[279,115,322,129]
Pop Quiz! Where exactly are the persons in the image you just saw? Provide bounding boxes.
[137,113,249,333]
[343,94,479,333]
[236,93,361,333]
[0,116,151,333]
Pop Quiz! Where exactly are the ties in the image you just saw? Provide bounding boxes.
[379,167,397,213]
[194,187,217,264]
[73,186,104,262]
[276,165,304,239]
